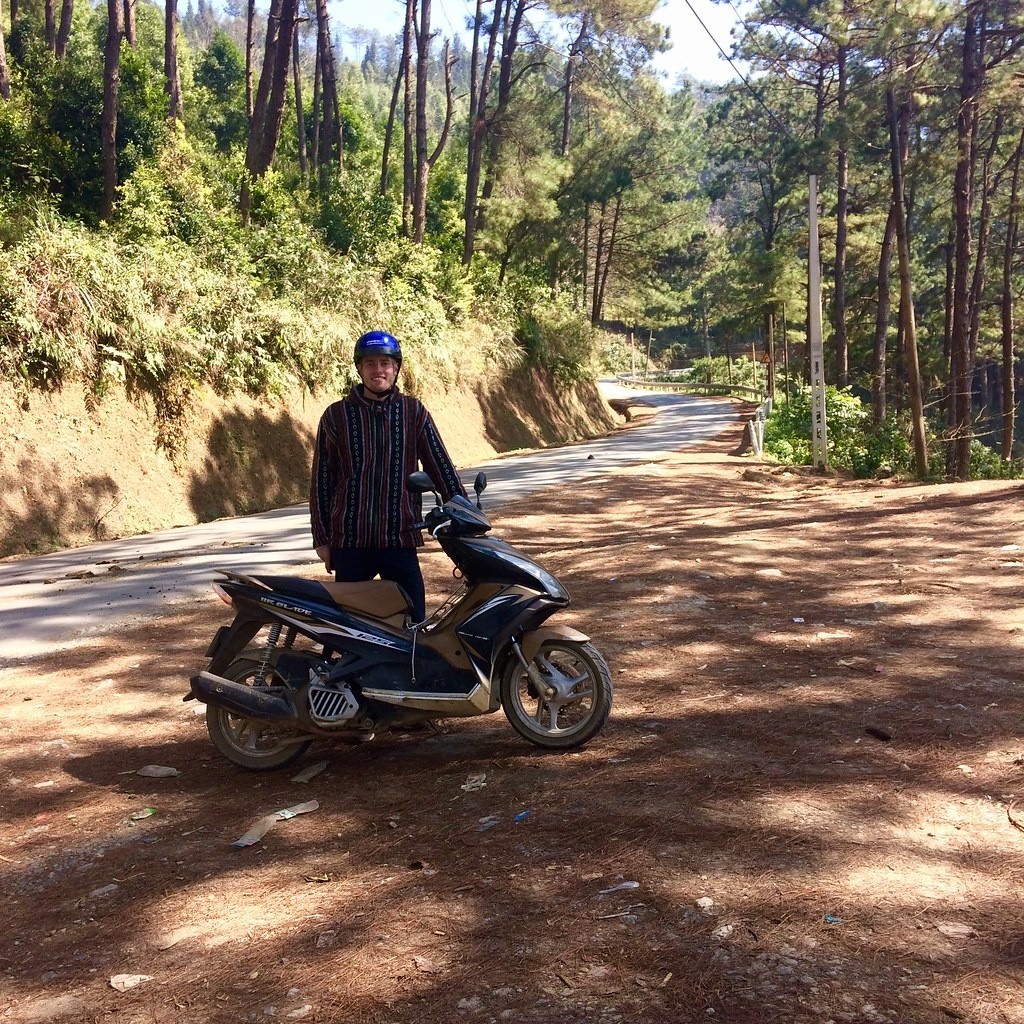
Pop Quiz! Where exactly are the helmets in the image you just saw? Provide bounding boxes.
[353,331,402,367]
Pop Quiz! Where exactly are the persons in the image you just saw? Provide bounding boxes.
[309,331,468,743]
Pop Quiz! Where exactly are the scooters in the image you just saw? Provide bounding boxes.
[184,470,617,770]
